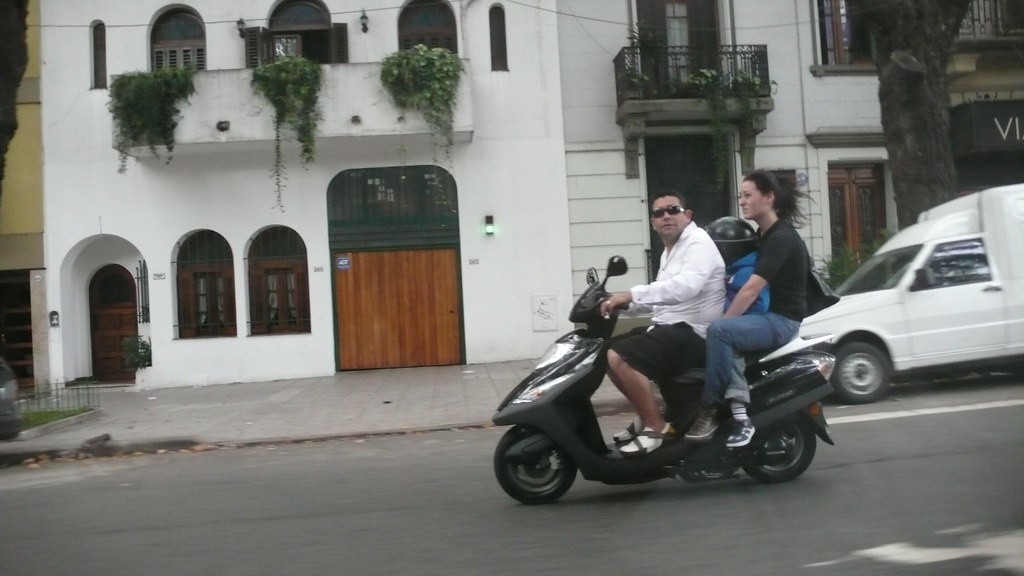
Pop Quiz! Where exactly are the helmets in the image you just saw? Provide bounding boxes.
[704,215,758,249]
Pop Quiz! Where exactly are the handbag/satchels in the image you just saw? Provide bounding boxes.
[807,265,840,319]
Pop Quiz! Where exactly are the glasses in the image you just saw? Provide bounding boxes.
[650,203,686,219]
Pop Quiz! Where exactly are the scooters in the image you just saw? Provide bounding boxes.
[492,255,837,504]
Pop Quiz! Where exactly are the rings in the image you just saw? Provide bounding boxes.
[607,301,612,304]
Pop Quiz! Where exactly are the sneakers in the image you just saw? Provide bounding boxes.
[725,419,756,447]
[685,407,722,441]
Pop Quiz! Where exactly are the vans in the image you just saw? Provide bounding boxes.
[798,183,1024,405]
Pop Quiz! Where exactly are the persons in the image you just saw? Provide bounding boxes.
[600,188,727,457]
[703,216,770,447]
[684,170,808,442]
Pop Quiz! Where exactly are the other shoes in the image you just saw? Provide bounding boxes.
[613,415,644,443]
[619,421,678,458]
